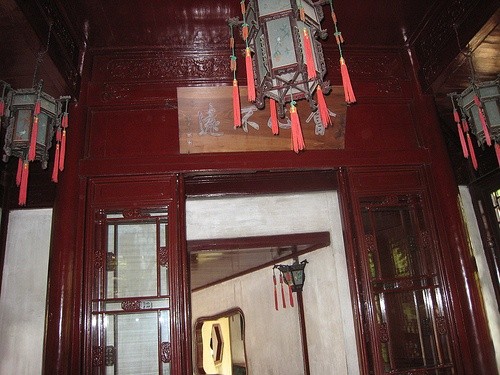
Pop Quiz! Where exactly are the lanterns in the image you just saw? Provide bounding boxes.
[226,0,357,154]
[0,78,71,204]
[449,78,500,169]
[271,258,309,311]
